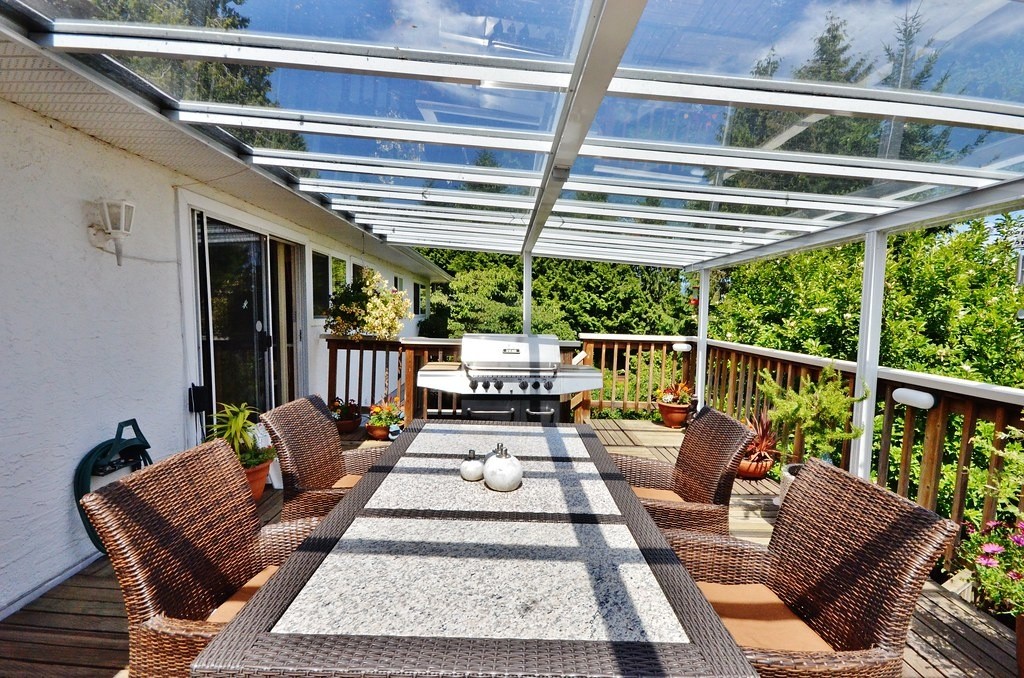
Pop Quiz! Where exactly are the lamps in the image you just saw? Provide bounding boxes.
[82,198,136,267]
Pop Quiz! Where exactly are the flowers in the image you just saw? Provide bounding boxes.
[957,519,1024,618]
[322,266,414,342]
[328,397,362,420]
[653,380,694,404]
[201,402,277,469]
[368,397,404,426]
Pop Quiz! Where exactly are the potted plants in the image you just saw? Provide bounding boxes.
[737,405,799,479]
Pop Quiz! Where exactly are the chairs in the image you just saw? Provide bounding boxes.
[81,438,328,678]
[608,407,759,536]
[660,457,961,678]
[259,393,391,523]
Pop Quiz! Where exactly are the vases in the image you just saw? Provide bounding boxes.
[657,400,691,427]
[1014,614,1024,678]
[779,463,805,513]
[335,420,362,434]
[243,458,274,501]
[371,426,388,439]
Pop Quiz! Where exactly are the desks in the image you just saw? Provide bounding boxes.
[189,419,764,678]
